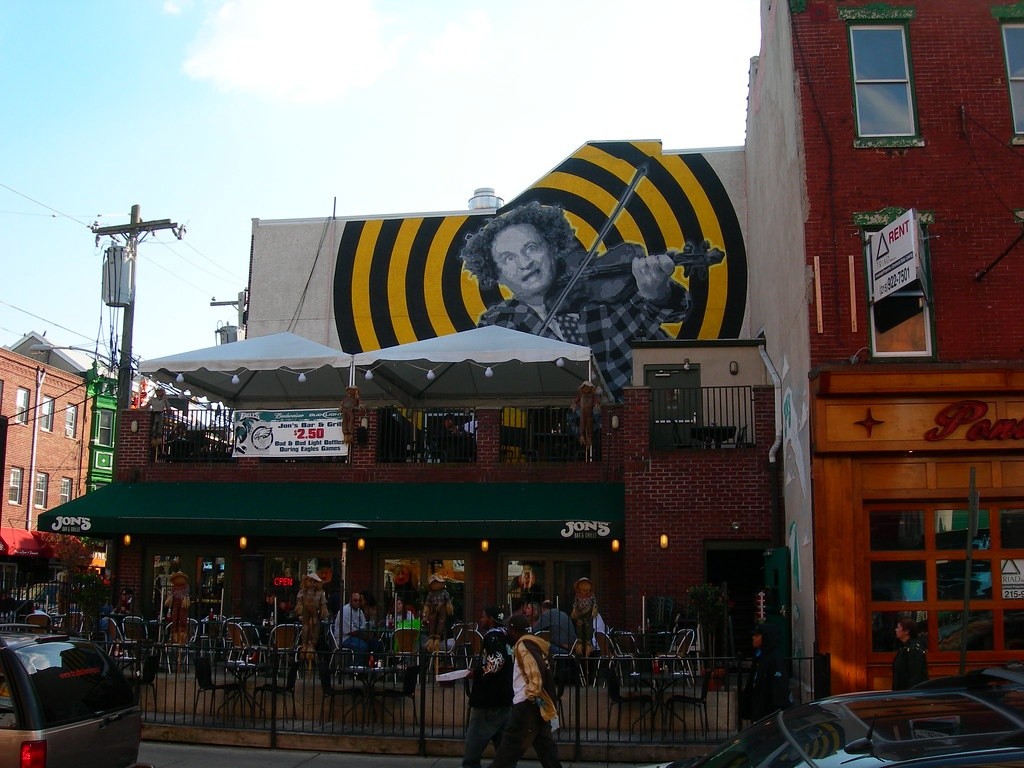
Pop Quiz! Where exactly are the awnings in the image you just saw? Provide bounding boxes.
[0,525,95,560]
[37,480,623,553]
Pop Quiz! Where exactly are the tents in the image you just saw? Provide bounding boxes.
[136,330,353,466]
[349,323,617,465]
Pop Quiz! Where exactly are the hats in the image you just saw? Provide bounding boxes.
[750,623,780,639]
[486,606,506,626]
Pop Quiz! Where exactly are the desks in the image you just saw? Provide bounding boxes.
[217,658,269,718]
[127,618,170,645]
[46,613,72,632]
[361,627,398,653]
[692,425,737,450]
[617,629,674,657]
[625,670,691,735]
[340,665,398,724]
[533,432,574,462]
[165,440,208,461]
[197,620,240,648]
[99,612,129,638]
[110,656,138,672]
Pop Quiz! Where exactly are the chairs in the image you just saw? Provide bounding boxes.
[671,422,694,450]
[22,600,712,737]
[734,425,746,448]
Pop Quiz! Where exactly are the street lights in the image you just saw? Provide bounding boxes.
[30,344,134,609]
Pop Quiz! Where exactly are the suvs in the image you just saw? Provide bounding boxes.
[646,661,1024,768]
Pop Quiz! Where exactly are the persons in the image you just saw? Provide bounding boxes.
[506,596,606,685]
[339,386,360,444]
[420,574,455,650]
[141,386,172,446]
[570,381,601,449]
[333,590,385,679]
[383,597,415,631]
[459,602,513,768]
[890,616,929,691]
[95,589,142,639]
[490,616,563,768]
[250,591,300,647]
[294,572,328,661]
[425,413,464,465]
[164,572,192,645]
[572,577,598,657]
[743,622,793,725]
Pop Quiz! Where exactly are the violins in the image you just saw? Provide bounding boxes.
[540,240,725,317]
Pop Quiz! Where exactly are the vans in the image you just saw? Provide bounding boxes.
[0,628,157,768]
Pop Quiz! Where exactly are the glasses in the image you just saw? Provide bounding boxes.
[504,624,513,631]
